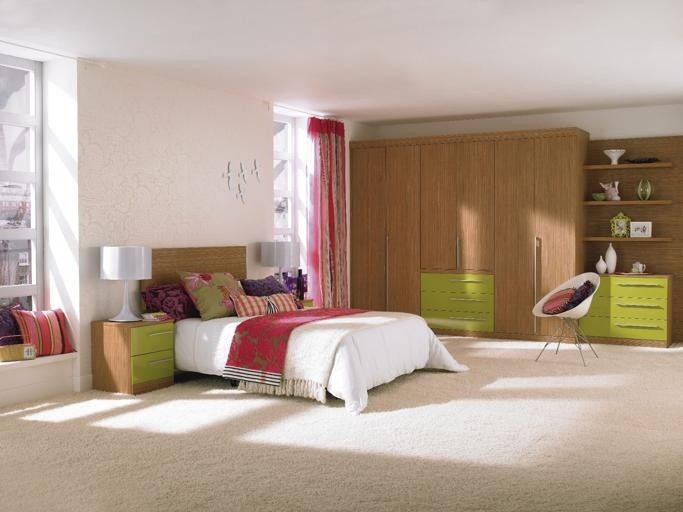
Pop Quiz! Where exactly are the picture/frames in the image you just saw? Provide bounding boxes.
[631,221,652,237]
[610,212,631,238]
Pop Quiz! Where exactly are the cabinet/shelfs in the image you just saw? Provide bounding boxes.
[578,273,673,349]
[493,126,589,343]
[420,273,494,338]
[419,132,493,274]
[349,138,420,316]
[583,161,673,242]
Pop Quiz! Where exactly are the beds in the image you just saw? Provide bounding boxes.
[141,246,469,413]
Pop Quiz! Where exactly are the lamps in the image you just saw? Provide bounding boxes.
[261,241,300,283]
[100,246,152,322]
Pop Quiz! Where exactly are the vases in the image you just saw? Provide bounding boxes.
[596,256,607,273]
[605,242,617,273]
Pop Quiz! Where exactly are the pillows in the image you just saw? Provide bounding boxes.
[543,287,575,315]
[11,309,73,356]
[0,298,28,345]
[229,294,298,318]
[566,281,595,310]
[239,276,290,296]
[175,272,247,321]
[140,284,193,322]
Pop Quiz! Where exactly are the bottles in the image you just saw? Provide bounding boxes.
[296,269,304,302]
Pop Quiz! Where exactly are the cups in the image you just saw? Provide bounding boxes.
[631,261,646,273]
[591,193,608,201]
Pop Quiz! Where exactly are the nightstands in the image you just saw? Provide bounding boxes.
[303,300,313,307]
[90,319,174,395]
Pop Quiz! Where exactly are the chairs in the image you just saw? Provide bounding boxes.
[532,272,600,367]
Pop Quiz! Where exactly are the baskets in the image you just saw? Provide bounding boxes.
[0,335,35,361]
